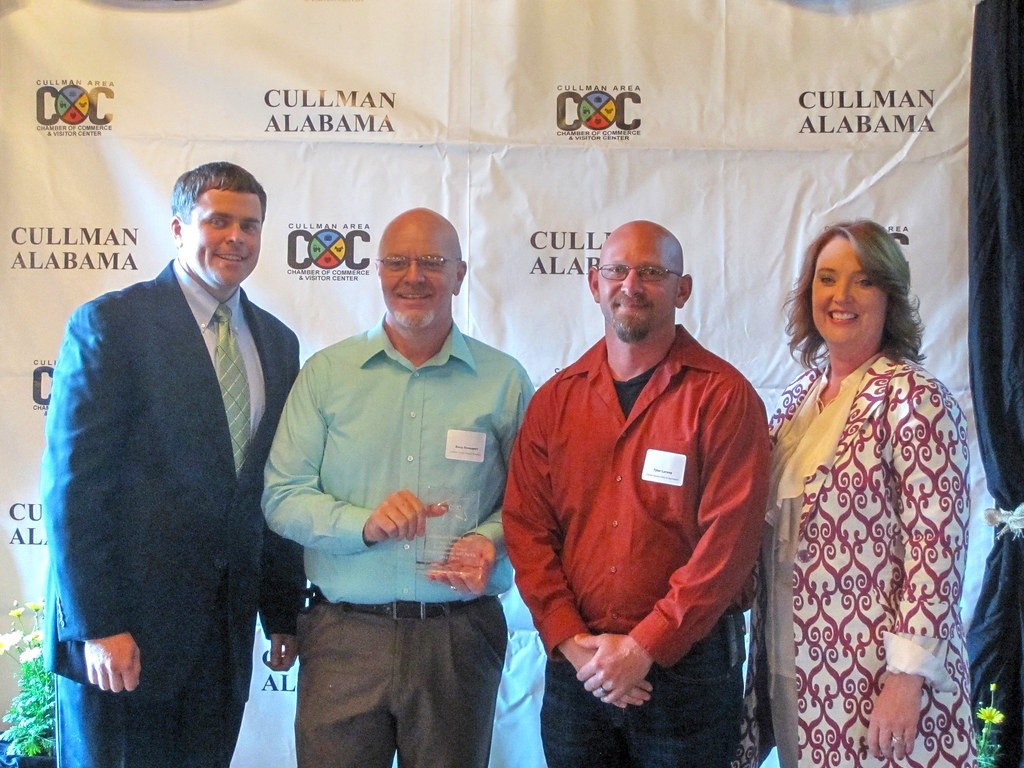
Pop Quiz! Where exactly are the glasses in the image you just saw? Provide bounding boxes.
[375,253,461,275]
[598,263,681,283]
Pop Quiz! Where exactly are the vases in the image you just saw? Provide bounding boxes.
[1,731,57,768]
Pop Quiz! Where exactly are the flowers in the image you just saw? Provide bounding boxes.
[973,682,1006,767]
[0,599,57,759]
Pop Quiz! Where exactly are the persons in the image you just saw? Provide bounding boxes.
[734,219,981,768]
[261,206,535,768]
[37,162,310,768]
[502,220,771,768]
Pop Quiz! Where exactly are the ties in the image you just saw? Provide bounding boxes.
[211,303,251,478]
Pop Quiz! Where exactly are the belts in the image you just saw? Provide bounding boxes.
[339,594,497,622]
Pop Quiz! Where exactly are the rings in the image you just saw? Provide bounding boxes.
[451,586,457,590]
[600,685,610,694]
[892,736,902,742]
